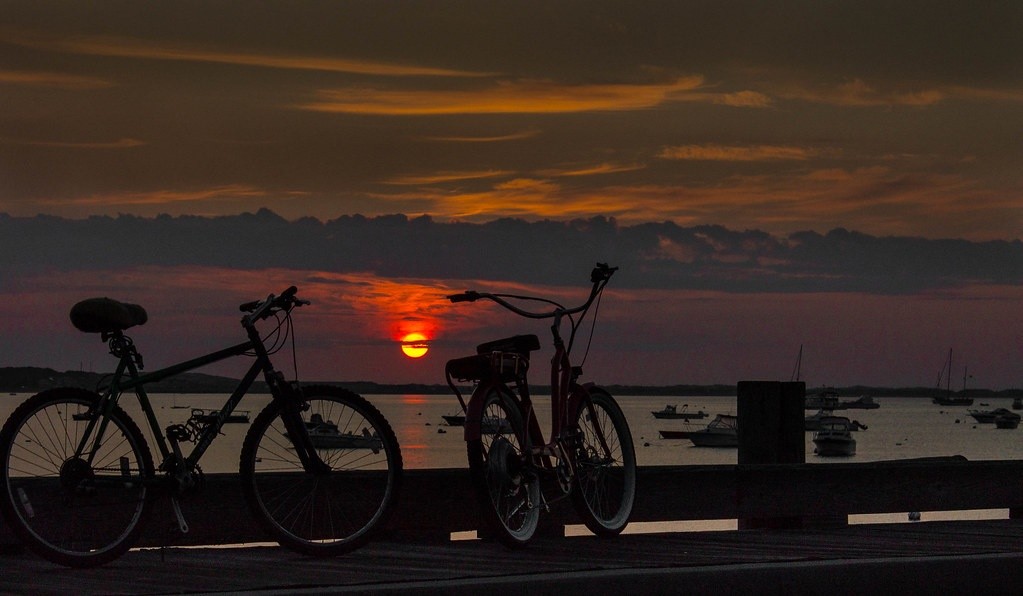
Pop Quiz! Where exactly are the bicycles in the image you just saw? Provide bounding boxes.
[446,263,636,548]
[1,286,403,569]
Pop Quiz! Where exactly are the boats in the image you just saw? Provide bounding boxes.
[72,412,92,421]
[968,408,1021,429]
[660,414,739,447]
[283,414,383,448]
[806,387,880,410]
[442,414,514,434]
[652,407,709,420]
[1011,398,1023,409]
[805,408,868,456]
[194,412,249,423]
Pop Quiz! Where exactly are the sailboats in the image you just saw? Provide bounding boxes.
[930,349,974,406]
